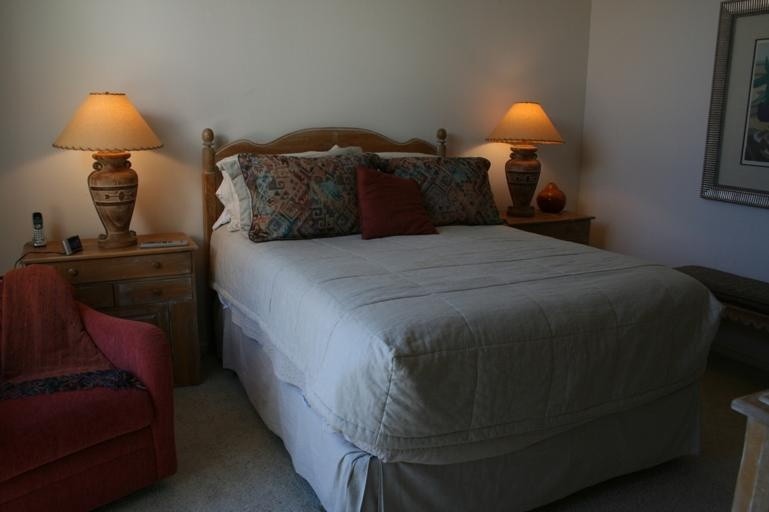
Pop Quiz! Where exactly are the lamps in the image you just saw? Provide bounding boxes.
[485,102,566,217]
[51,92,163,249]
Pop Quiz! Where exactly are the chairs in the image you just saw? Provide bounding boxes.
[1,264,178,511]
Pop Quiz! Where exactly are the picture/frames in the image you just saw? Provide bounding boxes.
[699,0,769,209]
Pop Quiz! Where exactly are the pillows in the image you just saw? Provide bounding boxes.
[213,144,504,243]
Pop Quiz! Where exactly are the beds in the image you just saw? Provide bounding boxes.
[200,123,712,511]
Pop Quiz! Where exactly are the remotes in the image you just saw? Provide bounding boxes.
[139,240,190,247]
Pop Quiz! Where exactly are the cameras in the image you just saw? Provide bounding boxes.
[62,234,83,255]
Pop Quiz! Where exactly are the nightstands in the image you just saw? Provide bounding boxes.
[20,231,203,388]
[497,209,595,246]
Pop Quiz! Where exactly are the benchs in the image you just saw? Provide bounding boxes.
[673,265,769,373]
